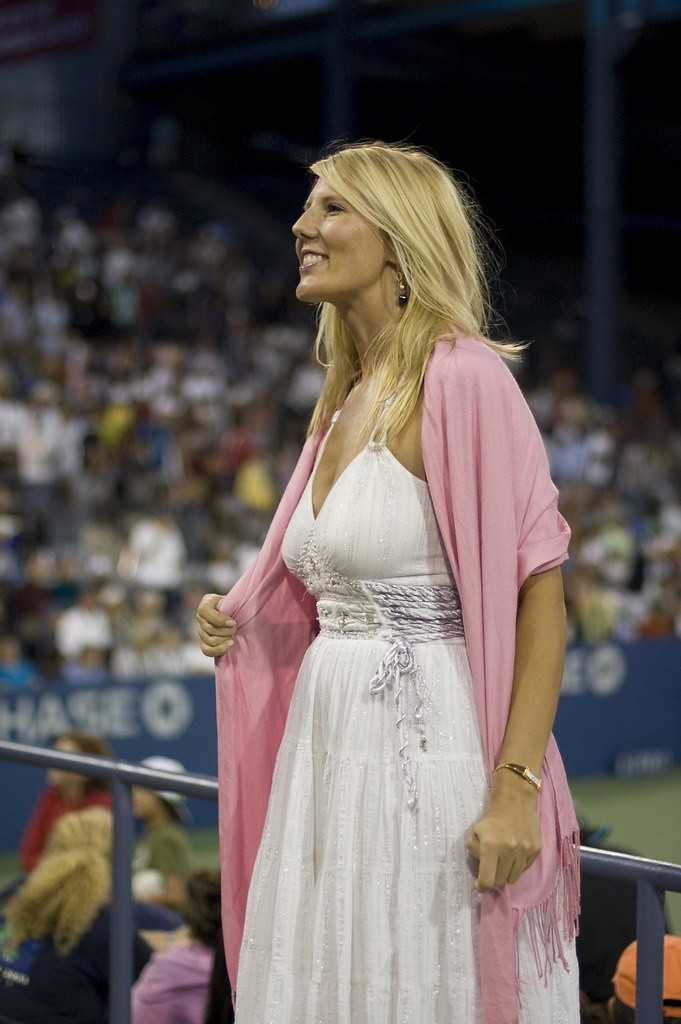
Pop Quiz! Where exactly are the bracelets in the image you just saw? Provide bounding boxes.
[493,763,542,790]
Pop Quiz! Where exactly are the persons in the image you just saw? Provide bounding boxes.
[574,819,681,1024]
[0,179,681,688]
[194,143,583,1024]
[0,734,236,1024]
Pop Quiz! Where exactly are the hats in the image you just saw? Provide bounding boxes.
[141,755,194,824]
[613,935,681,1017]
